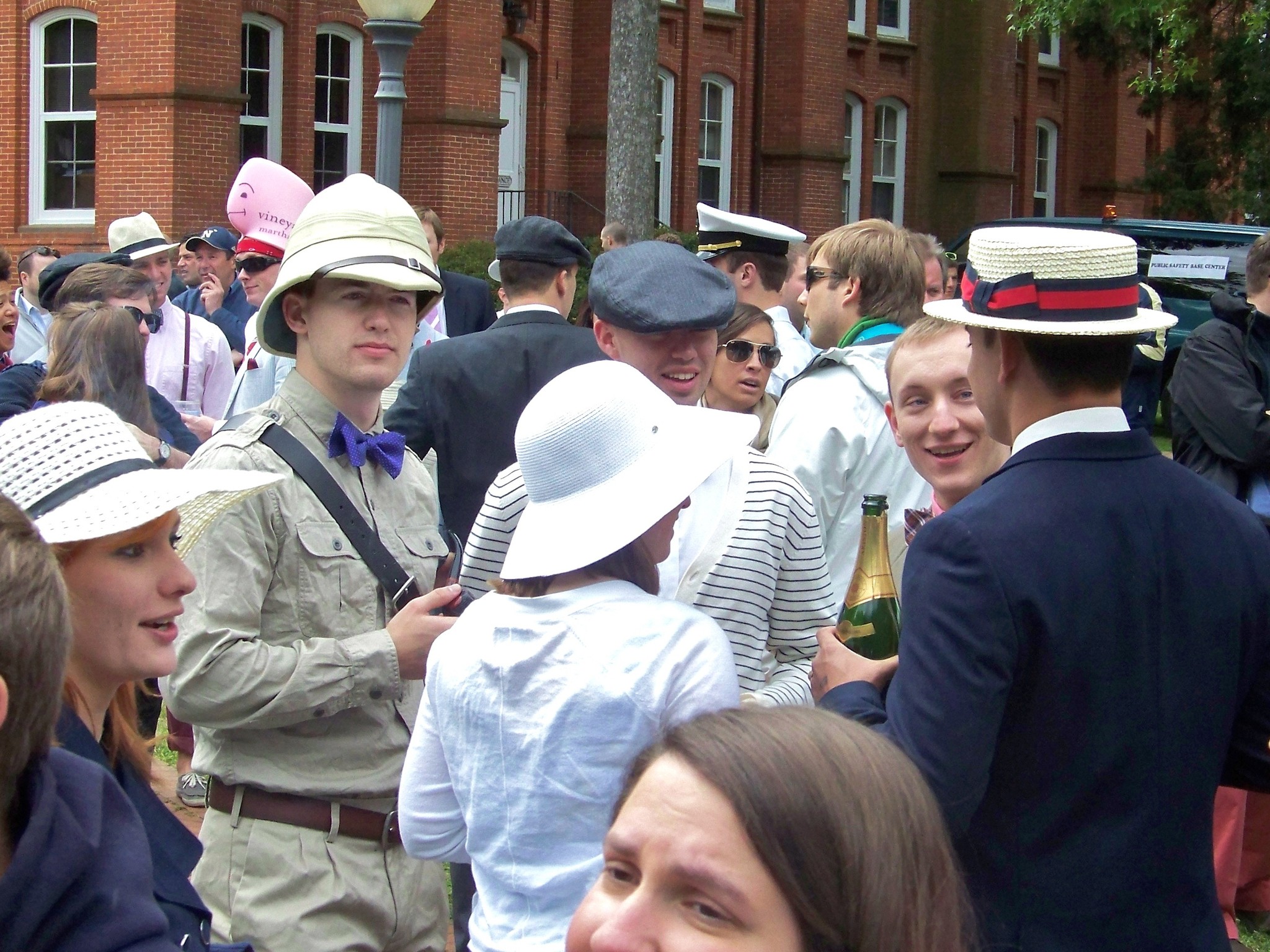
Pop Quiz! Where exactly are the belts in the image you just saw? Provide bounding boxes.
[208,777,400,848]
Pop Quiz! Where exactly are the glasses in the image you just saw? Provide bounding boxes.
[718,340,783,369]
[233,258,282,272]
[804,266,849,291]
[18,247,61,267]
[945,252,957,260]
[119,304,161,334]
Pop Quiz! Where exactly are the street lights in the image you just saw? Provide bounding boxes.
[356,0,436,194]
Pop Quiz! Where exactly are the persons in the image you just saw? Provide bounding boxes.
[0,174,1268,952]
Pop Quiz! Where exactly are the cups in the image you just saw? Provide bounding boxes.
[175,400,201,417]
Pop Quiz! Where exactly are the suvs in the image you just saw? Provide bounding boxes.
[943,205,1270,370]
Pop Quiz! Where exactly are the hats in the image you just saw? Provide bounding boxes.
[38,251,133,307]
[588,239,737,334]
[922,226,1180,335]
[695,201,807,260]
[107,212,180,258]
[497,360,762,581]
[255,171,446,358]
[0,402,295,560]
[186,225,238,251]
[495,215,591,265]
[226,156,315,251]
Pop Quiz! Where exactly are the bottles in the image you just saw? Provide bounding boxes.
[832,492,900,661]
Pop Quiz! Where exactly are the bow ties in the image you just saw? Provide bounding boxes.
[328,411,406,480]
[902,507,933,546]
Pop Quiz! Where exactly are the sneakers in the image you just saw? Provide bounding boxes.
[176,773,207,807]
